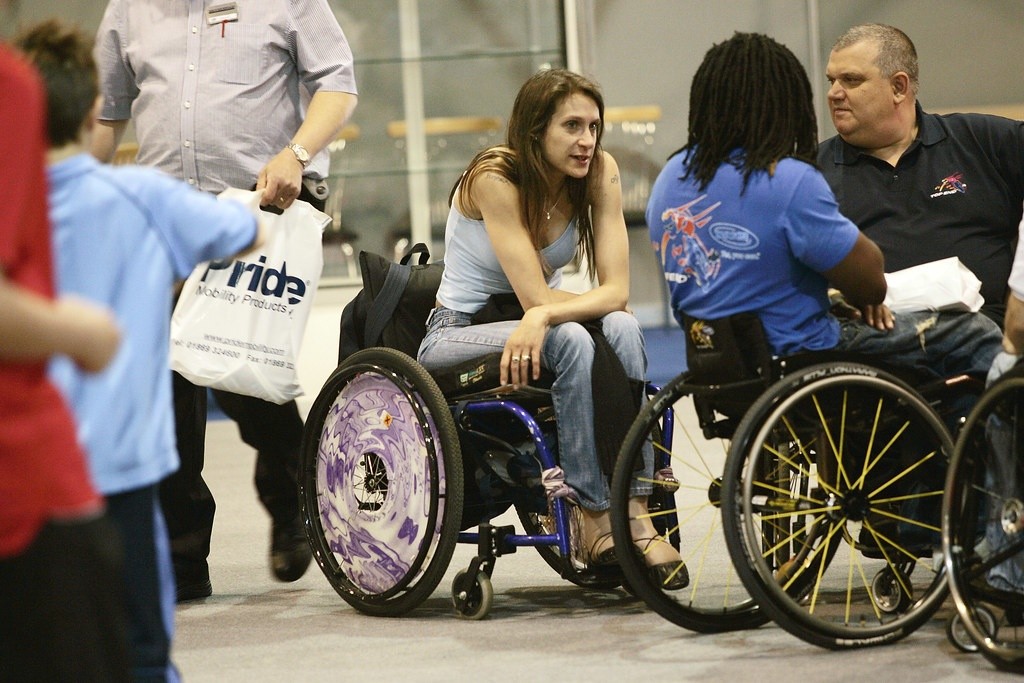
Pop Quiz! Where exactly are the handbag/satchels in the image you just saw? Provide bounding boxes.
[168,187,332,406]
[880,256,985,315]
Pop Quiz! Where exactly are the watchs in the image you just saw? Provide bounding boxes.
[285,141,312,170]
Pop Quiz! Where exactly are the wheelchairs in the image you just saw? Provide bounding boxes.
[296,243,681,621]
[608,308,1024,675]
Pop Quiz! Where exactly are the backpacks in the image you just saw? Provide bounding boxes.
[337,243,431,367]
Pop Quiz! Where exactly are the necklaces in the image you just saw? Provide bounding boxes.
[532,185,564,222]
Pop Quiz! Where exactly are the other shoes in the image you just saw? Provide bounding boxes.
[271,514,313,582]
[633,534,690,591]
[588,531,645,567]
[176,577,212,602]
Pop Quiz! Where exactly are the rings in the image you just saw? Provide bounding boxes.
[512,357,520,363]
[521,354,531,362]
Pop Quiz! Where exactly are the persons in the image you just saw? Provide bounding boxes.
[0,41,173,683]
[9,18,258,682]
[640,30,1024,628]
[802,23,1024,306]
[417,70,692,593]
[969,221,1024,608]
[83,1,358,605]
